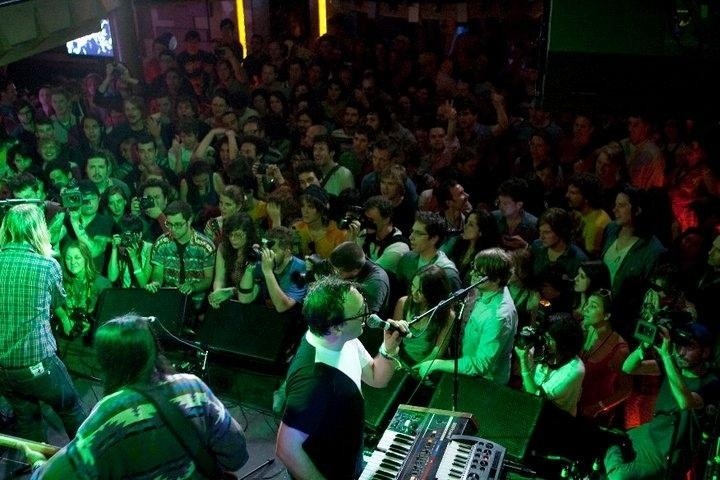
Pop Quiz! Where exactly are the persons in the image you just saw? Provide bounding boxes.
[1,17,719,479]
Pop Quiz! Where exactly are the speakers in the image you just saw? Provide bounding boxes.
[91,288,187,346]
[428,372,547,462]
[200,299,292,364]
[360,369,420,433]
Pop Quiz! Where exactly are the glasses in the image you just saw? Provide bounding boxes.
[408,228,433,236]
[469,261,487,277]
[164,219,189,229]
[409,284,425,297]
[335,301,371,327]
[595,287,617,303]
[650,282,664,293]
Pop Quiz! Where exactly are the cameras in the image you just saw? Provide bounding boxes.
[254,164,268,175]
[337,204,364,231]
[244,240,275,263]
[61,190,83,211]
[139,196,155,210]
[633,319,692,348]
[118,229,144,258]
[289,254,335,290]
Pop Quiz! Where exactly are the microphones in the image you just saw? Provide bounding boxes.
[366,313,413,340]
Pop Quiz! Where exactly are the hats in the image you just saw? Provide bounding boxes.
[296,182,334,204]
[672,322,714,348]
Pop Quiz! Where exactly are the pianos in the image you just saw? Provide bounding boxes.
[356,402,507,480]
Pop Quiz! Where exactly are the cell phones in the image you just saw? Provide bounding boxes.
[512,299,552,357]
